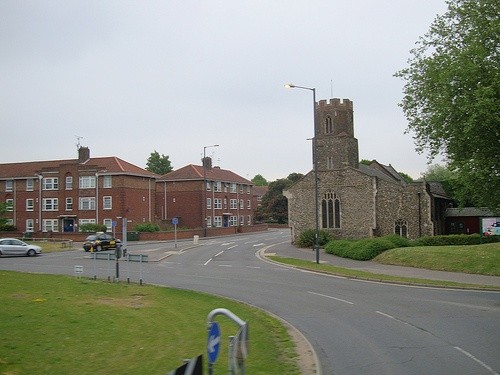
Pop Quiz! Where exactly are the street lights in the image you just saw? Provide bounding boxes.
[203,144,221,237]
[284,83,320,265]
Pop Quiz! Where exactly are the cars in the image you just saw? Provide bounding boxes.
[0,237,43,257]
[82,232,122,252]
